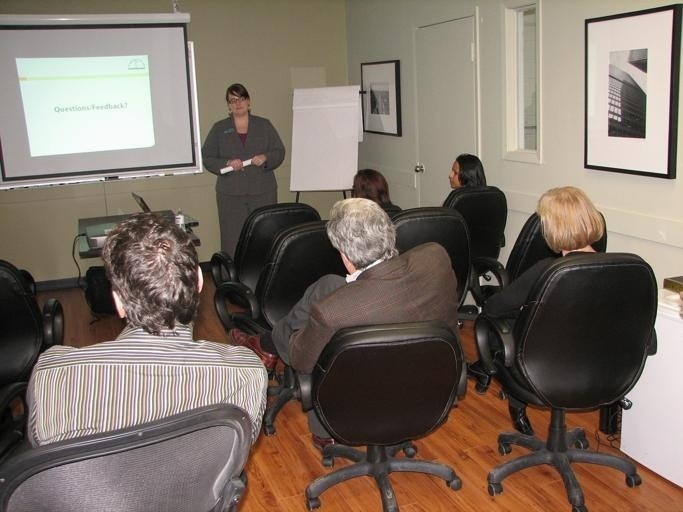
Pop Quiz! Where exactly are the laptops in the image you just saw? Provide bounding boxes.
[130,190,200,229]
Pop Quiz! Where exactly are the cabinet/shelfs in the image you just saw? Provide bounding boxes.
[620,271,683,489]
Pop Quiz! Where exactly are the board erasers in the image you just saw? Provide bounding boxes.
[104,176,119,181]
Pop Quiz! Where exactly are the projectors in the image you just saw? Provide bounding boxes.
[82,222,125,249]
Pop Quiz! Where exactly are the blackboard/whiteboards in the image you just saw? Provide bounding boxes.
[1,42,204,192]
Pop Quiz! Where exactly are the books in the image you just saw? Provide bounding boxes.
[288,84,364,193]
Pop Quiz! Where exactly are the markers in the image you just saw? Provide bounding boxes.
[165,173,172,176]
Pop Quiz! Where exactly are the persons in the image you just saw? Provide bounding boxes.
[201,83,286,283]
[228,197,459,457]
[24,210,269,450]
[350,168,403,220]
[447,154,487,190]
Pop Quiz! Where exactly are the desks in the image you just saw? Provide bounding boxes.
[78,209,201,260]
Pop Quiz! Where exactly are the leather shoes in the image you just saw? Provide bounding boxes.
[229,327,277,374]
[311,434,336,456]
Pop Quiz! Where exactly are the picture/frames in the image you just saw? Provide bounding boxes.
[361,61,403,138]
[584,3,682,180]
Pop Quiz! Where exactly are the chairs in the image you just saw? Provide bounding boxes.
[392,207,472,311]
[0,259,65,427]
[214,219,349,438]
[290,320,467,512]
[444,185,507,328]
[210,203,322,310]
[473,252,658,511]
[0,405,252,512]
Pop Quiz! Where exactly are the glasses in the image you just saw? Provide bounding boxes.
[228,96,246,105]
